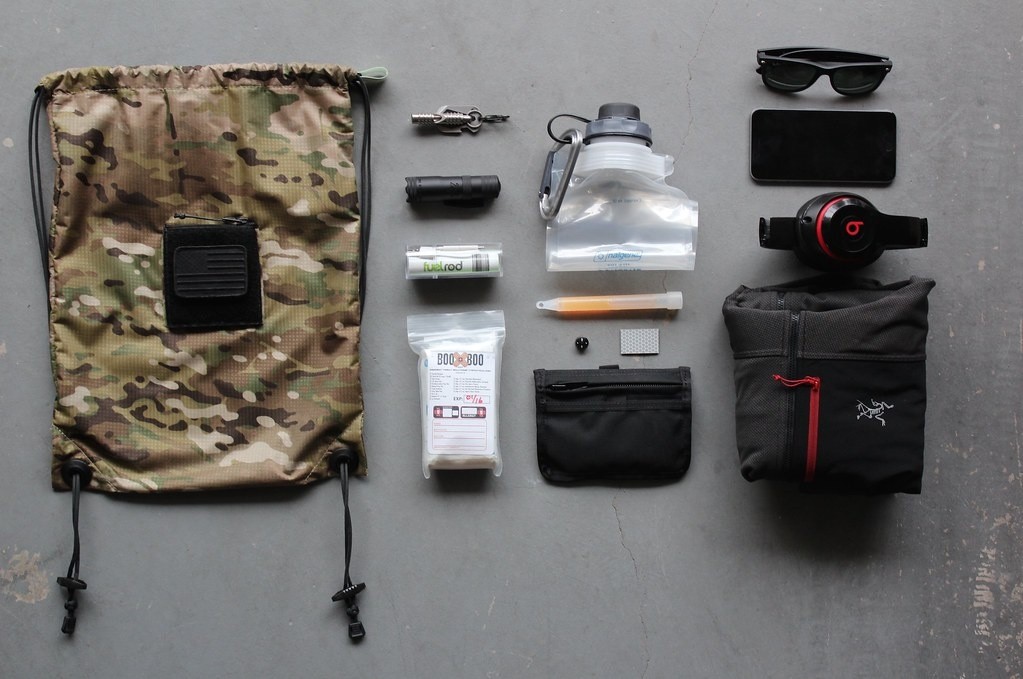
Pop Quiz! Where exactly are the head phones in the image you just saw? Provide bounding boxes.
[759,191,928,275]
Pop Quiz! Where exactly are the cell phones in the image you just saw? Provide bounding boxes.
[750,108,897,185]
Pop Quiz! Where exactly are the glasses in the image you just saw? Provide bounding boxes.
[755,47,893,98]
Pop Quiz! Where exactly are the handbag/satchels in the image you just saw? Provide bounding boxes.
[27,65,387,500]
[722,276,938,506]
